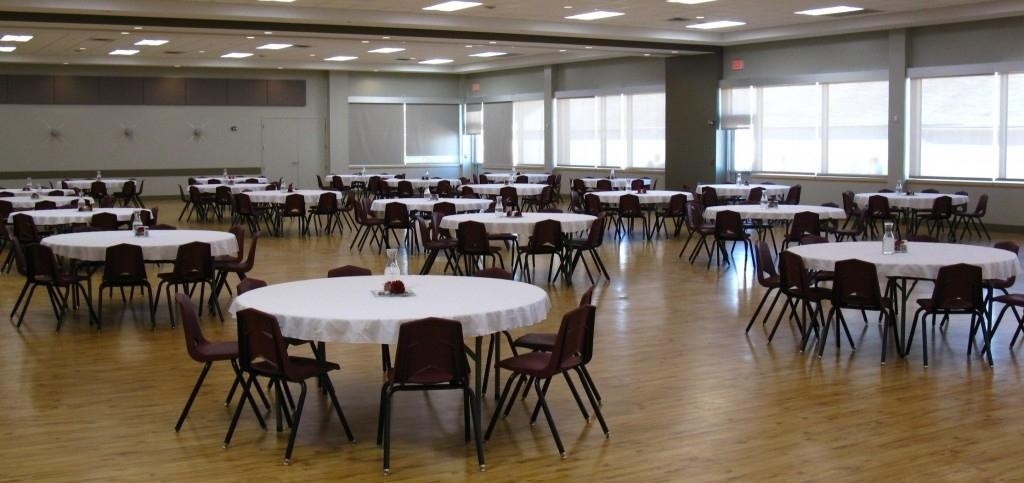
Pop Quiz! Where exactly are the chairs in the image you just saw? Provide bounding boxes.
[316,176,335,190]
[438,180,450,197]
[514,176,527,207]
[379,202,419,255]
[746,241,827,331]
[366,176,380,191]
[324,191,358,232]
[0,200,21,254]
[905,263,994,367]
[460,177,470,185]
[597,179,612,191]
[212,186,243,224]
[818,258,903,365]
[689,203,731,266]
[766,252,857,354]
[62,181,80,196]
[982,294,1024,359]
[304,192,343,235]
[152,241,224,329]
[223,308,358,466]
[319,265,389,395]
[872,189,908,236]
[731,201,778,256]
[0,192,14,197]
[397,181,413,198]
[513,220,571,288]
[130,224,178,301]
[915,195,956,242]
[189,226,244,304]
[245,179,259,183]
[9,237,68,319]
[836,191,879,241]
[416,216,468,275]
[819,202,839,241]
[570,179,592,210]
[130,210,151,229]
[484,203,495,213]
[16,244,101,333]
[173,293,271,430]
[521,186,552,211]
[376,317,485,476]
[208,179,219,183]
[570,190,585,213]
[127,180,145,208]
[463,267,528,396]
[948,194,991,241]
[246,178,258,184]
[770,185,801,224]
[929,191,972,237]
[831,208,868,242]
[225,278,326,411]
[394,174,405,179]
[546,175,560,208]
[781,212,829,252]
[614,194,651,241]
[70,199,78,208]
[84,181,107,208]
[914,189,944,235]
[423,213,463,275]
[679,204,726,260]
[479,175,494,184]
[879,235,949,322]
[977,241,1024,334]
[98,243,155,330]
[523,208,564,274]
[940,242,1024,333]
[568,178,574,210]
[368,176,383,202]
[358,198,400,246]
[454,220,504,275]
[741,187,766,223]
[198,233,257,317]
[500,187,520,212]
[863,195,901,240]
[188,177,202,185]
[631,202,658,239]
[487,305,609,460]
[178,185,218,222]
[231,193,275,236]
[377,180,398,198]
[91,212,118,230]
[429,177,442,199]
[113,181,140,208]
[187,186,221,223]
[35,201,56,237]
[631,179,644,191]
[333,176,351,204]
[555,174,564,205]
[585,193,626,239]
[49,191,64,196]
[74,227,126,305]
[350,181,367,201]
[350,200,390,251]
[514,286,602,426]
[721,182,746,205]
[789,236,868,326]
[424,202,455,254]
[261,185,276,220]
[553,218,610,283]
[1,213,43,273]
[707,209,755,270]
[274,194,311,238]
[651,194,692,240]
[701,187,729,218]
[462,186,480,198]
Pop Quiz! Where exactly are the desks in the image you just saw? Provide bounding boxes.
[703,205,846,262]
[57,180,136,196]
[187,184,285,219]
[5,209,153,240]
[325,175,396,191]
[40,230,239,325]
[786,241,1020,353]
[458,184,549,211]
[229,275,552,423]
[571,177,652,187]
[584,191,694,239]
[195,178,268,184]
[0,196,94,211]
[245,190,342,233]
[854,192,969,239]
[485,174,549,204]
[383,179,462,197]
[371,199,493,212]
[0,189,76,196]
[696,185,791,205]
[439,214,597,285]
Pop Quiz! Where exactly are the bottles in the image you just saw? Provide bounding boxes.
[424,168,429,179]
[610,168,615,180]
[25,176,33,189]
[760,190,768,211]
[882,222,895,255]
[280,178,288,193]
[895,179,902,196]
[510,167,517,176]
[508,173,514,188]
[78,192,85,210]
[362,168,366,177]
[97,170,101,181]
[424,182,430,201]
[133,211,143,236]
[495,196,503,218]
[36,184,43,199]
[625,176,631,194]
[222,168,228,179]
[736,173,742,187]
[384,249,400,283]
